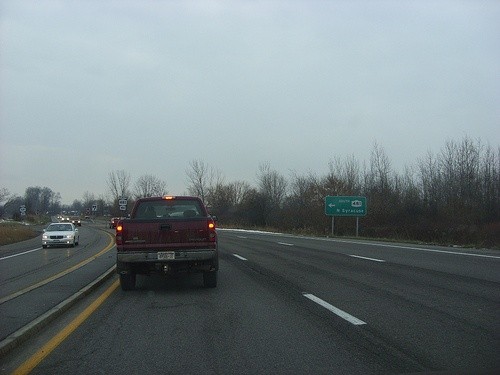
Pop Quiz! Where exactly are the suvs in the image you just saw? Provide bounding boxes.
[109,216,121,229]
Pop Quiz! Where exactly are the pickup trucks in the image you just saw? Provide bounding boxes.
[114,195,219,292]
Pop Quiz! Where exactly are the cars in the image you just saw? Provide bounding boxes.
[0,219,5,224]
[57,215,63,219]
[18,222,35,226]
[68,211,79,216]
[122,213,130,220]
[62,217,70,222]
[85,214,90,219]
[104,213,112,217]
[41,223,79,248]
[6,219,14,222]
[71,218,81,226]
[62,211,65,214]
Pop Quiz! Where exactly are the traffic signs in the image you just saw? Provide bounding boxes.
[324,195,367,217]
